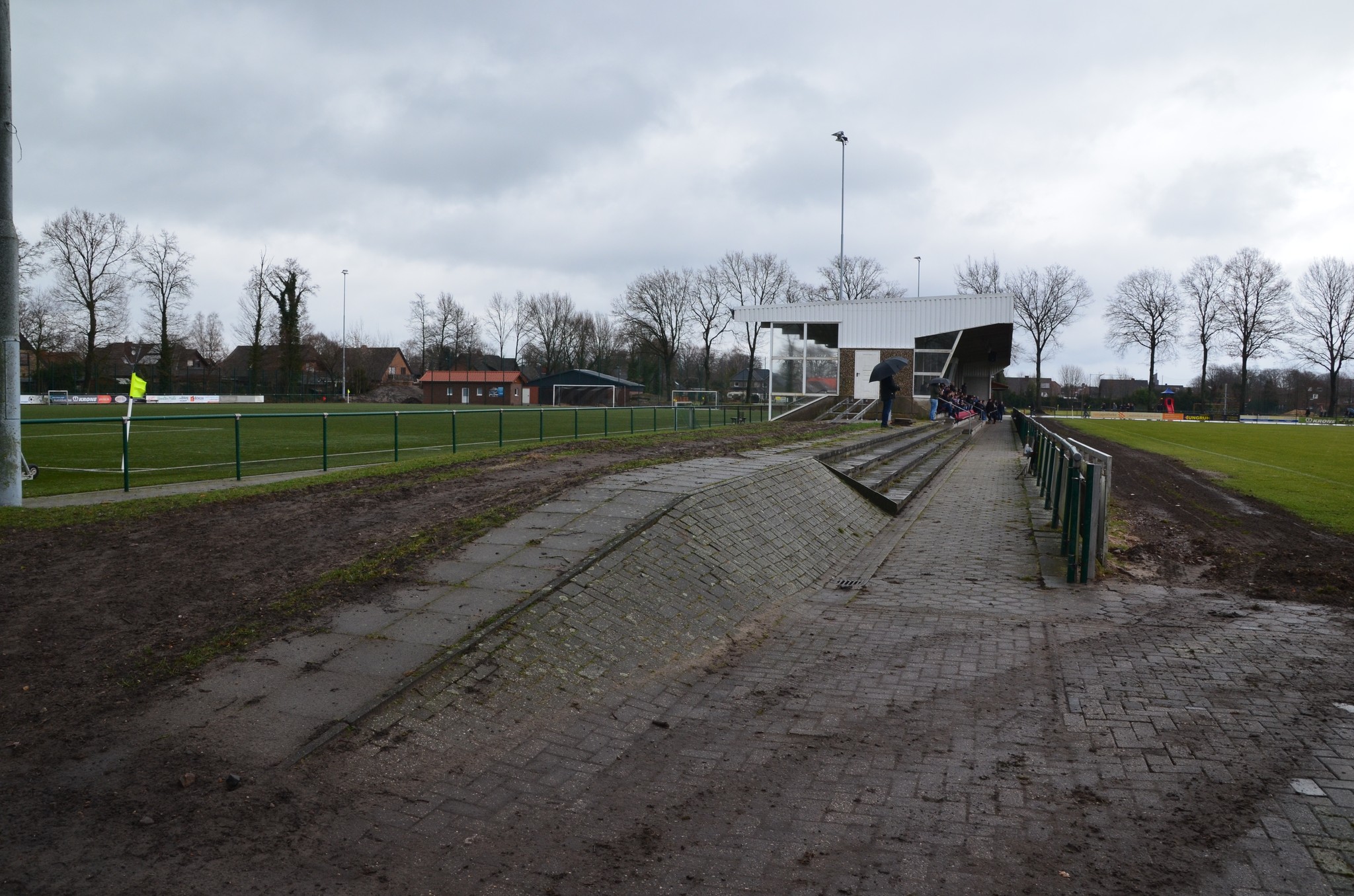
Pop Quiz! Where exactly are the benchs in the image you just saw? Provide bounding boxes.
[730,417,747,424]
[937,406,979,421]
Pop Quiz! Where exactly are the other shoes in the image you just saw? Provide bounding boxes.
[992,422,996,424]
[979,418,981,420]
[999,420,1000,421]
[1001,421,1002,422]
[930,420,938,422]
[1035,414,1036,417]
[1030,417,1032,417]
[988,419,991,421]
[950,416,955,420]
[881,425,893,429]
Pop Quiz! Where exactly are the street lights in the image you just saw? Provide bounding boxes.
[914,256,921,297]
[832,130,849,301]
[341,269,348,399]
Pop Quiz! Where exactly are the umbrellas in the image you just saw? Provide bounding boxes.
[927,378,952,384]
[1348,408,1354,413]
[869,356,909,383]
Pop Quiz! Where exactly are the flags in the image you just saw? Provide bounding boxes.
[130,372,147,398]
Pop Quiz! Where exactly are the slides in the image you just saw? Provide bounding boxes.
[1167,404,1174,414]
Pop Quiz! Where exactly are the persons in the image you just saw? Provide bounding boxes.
[1113,402,1117,411]
[959,394,979,411]
[974,400,986,420]
[962,383,967,394]
[1102,402,1106,411]
[986,399,1004,424]
[1305,408,1310,417]
[1158,403,1163,411]
[880,376,900,427]
[1320,409,1326,417]
[1120,403,1134,412]
[1083,403,1090,418]
[921,381,963,420]
[1030,402,1036,417]
[1344,409,1352,418]
[929,383,943,422]
